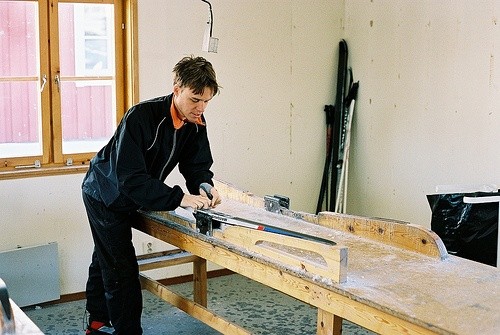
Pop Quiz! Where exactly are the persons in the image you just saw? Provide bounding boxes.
[81,54,223,335]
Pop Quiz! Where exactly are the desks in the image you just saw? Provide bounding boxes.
[131,193,500,335]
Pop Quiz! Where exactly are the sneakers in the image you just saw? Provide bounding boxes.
[85,321,117,335]
